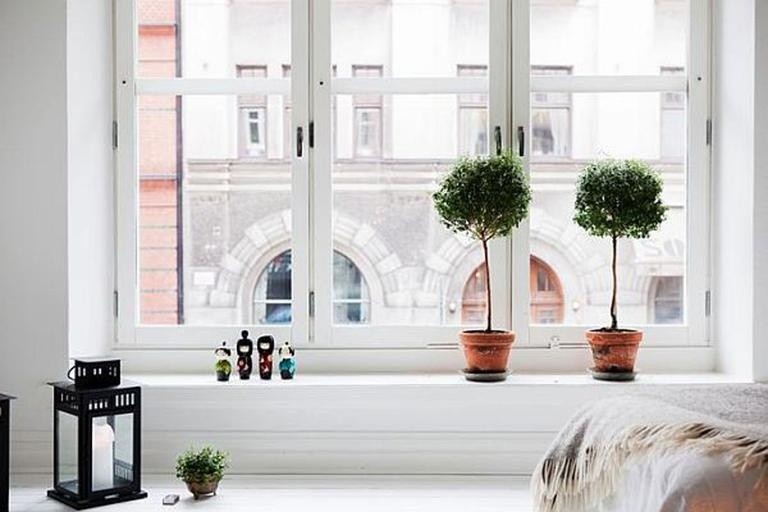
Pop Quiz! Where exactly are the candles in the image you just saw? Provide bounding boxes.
[93,422,117,490]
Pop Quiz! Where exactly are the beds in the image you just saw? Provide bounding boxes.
[535,385,768,512]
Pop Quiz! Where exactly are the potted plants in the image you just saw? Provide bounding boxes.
[570,154,666,381]
[178,448,233,499]
[433,149,533,383]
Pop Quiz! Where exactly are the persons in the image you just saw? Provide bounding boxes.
[236,330,253,380]
[256,334,275,380]
[278,341,296,380]
[214,339,234,381]
